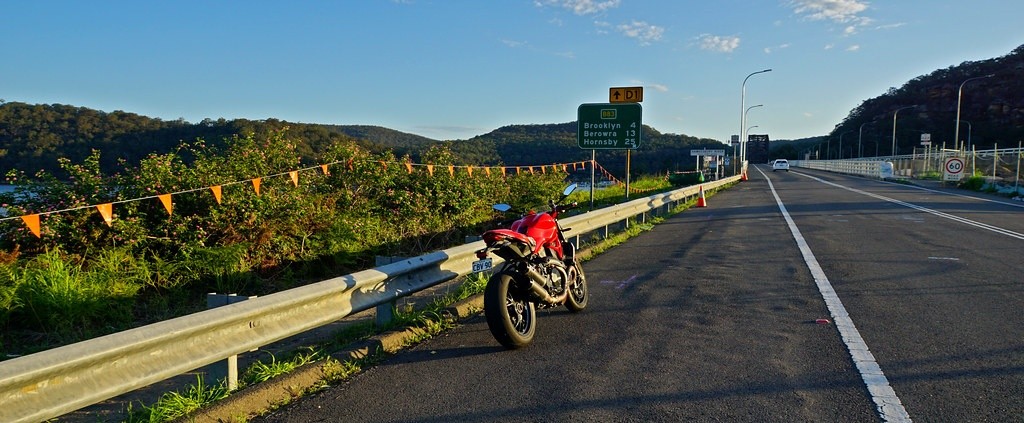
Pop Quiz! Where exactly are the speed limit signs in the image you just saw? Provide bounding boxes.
[944,158,964,181]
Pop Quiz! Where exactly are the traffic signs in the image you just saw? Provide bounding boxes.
[578,103,642,149]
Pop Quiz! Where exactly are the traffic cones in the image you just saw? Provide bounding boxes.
[741,171,748,181]
[695,186,707,207]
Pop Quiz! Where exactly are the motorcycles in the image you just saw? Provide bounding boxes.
[471,182,589,350]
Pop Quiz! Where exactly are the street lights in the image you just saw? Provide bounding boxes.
[891,105,917,155]
[746,126,758,158]
[858,122,868,158]
[954,73,995,158]
[740,68,773,168]
[797,129,854,160]
[743,105,763,160]
